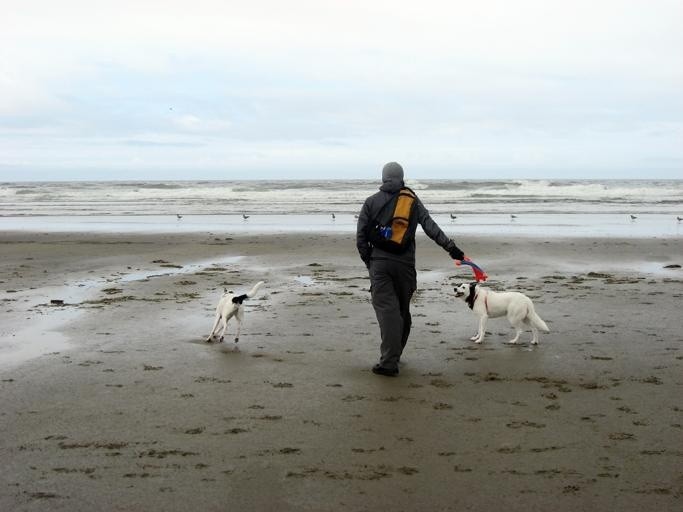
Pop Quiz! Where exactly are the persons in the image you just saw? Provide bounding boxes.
[355,162,464,375]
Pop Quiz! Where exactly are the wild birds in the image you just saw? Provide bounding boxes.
[243,214,249,218]
[177,214,183,218]
[630,215,637,219]
[451,213,456,220]
[331,213,335,219]
[677,217,683,221]
[511,214,517,218]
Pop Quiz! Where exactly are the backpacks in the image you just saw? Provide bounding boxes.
[366,186,419,256]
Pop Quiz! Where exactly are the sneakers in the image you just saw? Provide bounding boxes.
[372,355,402,378]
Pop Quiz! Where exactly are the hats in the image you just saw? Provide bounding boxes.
[381,161,404,183]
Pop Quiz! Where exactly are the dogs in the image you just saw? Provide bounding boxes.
[205,280,264,342]
[454,283,550,345]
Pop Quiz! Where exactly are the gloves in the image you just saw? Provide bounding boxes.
[450,246,466,262]
